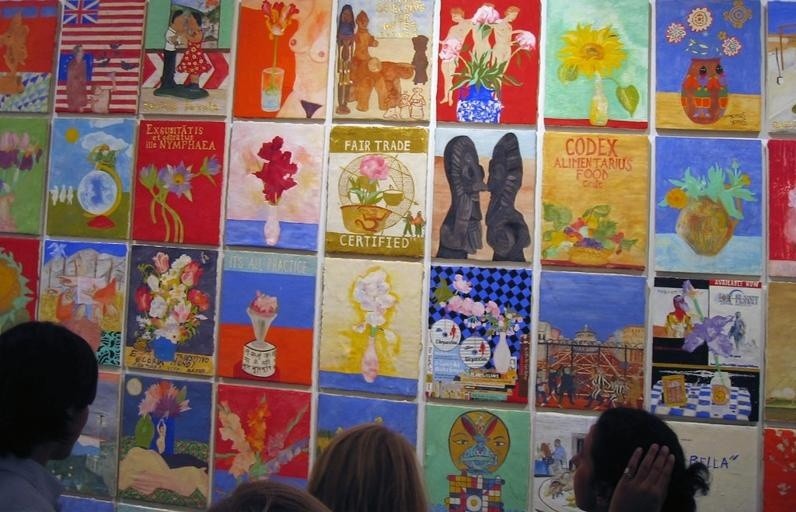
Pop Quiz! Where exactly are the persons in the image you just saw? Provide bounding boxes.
[336,2,357,114]
[551,437,565,463]
[307,422,430,511]
[490,5,524,88]
[65,44,88,112]
[207,478,336,512]
[178,11,211,88]
[438,6,474,106]
[50,258,121,324]
[575,404,711,511]
[733,310,748,358]
[159,8,185,88]
[470,1,496,65]
[1,319,99,512]
[239,0,332,120]
[350,8,380,62]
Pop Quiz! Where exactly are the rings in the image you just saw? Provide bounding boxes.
[623,467,635,478]
